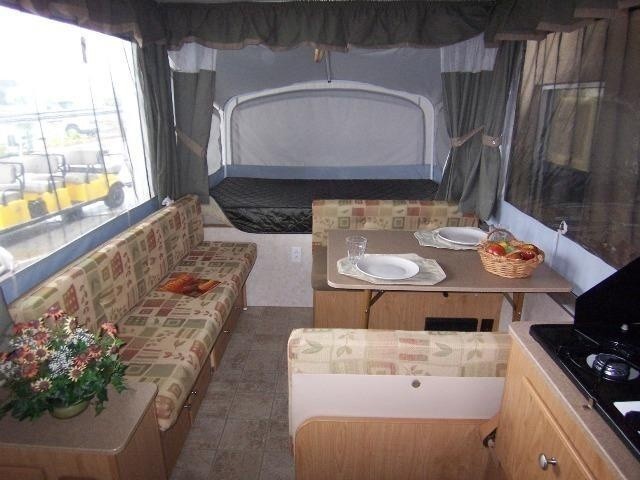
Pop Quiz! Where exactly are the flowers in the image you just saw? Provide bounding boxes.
[0,307,129,424]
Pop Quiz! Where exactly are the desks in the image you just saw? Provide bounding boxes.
[492,320,639,479]
[327,228,570,328]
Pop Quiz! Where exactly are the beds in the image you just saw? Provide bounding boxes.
[200,80,442,308]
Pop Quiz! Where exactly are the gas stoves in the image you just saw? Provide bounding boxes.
[530,323,640,463]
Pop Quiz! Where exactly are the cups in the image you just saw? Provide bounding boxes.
[344,235,368,264]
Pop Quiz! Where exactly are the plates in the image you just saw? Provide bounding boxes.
[355,252,421,280]
[436,226,489,246]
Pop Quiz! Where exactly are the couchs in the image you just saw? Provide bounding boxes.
[0,194,258,480]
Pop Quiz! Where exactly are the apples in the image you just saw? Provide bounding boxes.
[488,240,538,260]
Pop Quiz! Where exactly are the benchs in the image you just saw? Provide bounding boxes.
[311,198,505,328]
[0,148,123,204]
[288,328,511,480]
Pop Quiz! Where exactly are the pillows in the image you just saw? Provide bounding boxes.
[8,193,204,336]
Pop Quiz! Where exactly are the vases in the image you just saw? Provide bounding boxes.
[52,400,89,421]
